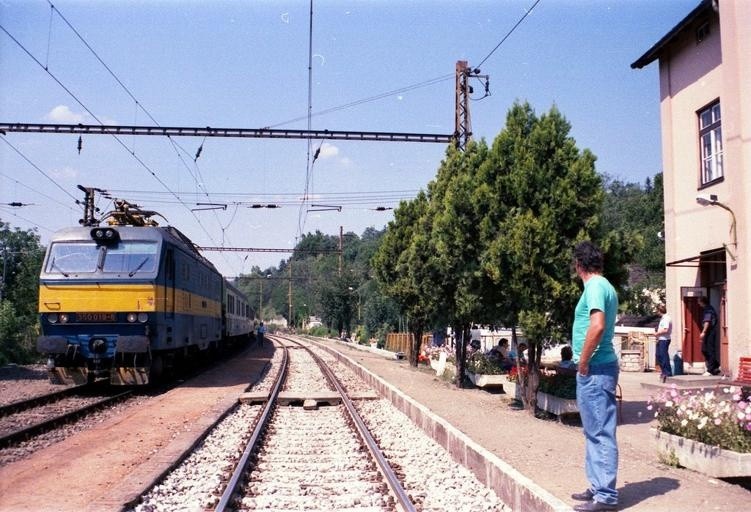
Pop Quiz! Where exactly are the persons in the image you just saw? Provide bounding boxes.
[558,343,575,370]
[256,322,264,349]
[696,294,721,378]
[572,238,623,512]
[508,343,530,366]
[487,332,516,370]
[652,303,674,379]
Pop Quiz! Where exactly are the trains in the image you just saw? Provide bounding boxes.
[36,185,256,386]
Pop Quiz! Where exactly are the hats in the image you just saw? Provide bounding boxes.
[470,340,481,349]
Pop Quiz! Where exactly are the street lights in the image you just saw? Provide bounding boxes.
[348,286,361,331]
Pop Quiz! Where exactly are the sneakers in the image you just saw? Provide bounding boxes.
[657,370,672,378]
[702,371,711,376]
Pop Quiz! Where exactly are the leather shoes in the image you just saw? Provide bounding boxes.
[571,488,618,512]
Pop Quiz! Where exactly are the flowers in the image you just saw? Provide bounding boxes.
[638,384,751,454]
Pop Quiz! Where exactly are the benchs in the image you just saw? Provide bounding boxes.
[718,357,751,402]
[554,365,623,424]
